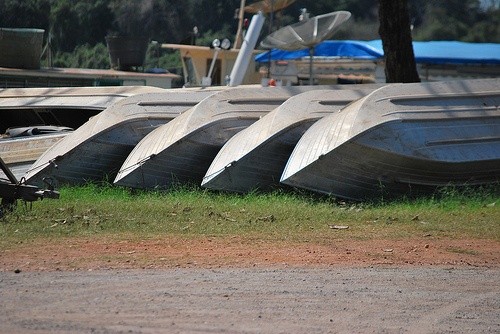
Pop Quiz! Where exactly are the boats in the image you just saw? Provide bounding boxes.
[2,0,500,203]
